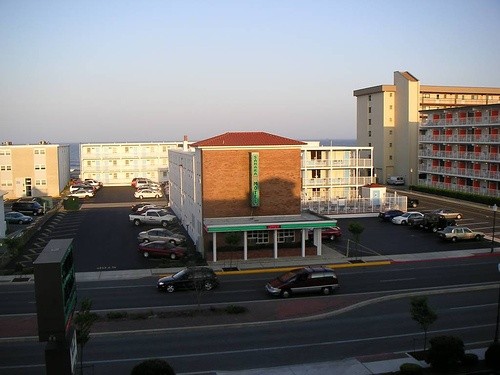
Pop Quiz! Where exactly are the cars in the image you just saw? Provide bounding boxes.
[391,211,424,226]
[4,211,34,226]
[156,266,219,295]
[136,228,188,247]
[407,207,464,234]
[136,240,188,261]
[436,227,486,244]
[265,265,341,299]
[308,226,344,242]
[378,210,404,223]
[66,179,103,201]
[131,177,169,200]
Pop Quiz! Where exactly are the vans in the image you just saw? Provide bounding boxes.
[11,201,47,217]
[387,175,405,187]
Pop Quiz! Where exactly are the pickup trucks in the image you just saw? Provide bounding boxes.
[128,203,177,229]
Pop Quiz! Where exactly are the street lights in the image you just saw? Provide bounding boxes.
[490,204,498,253]
